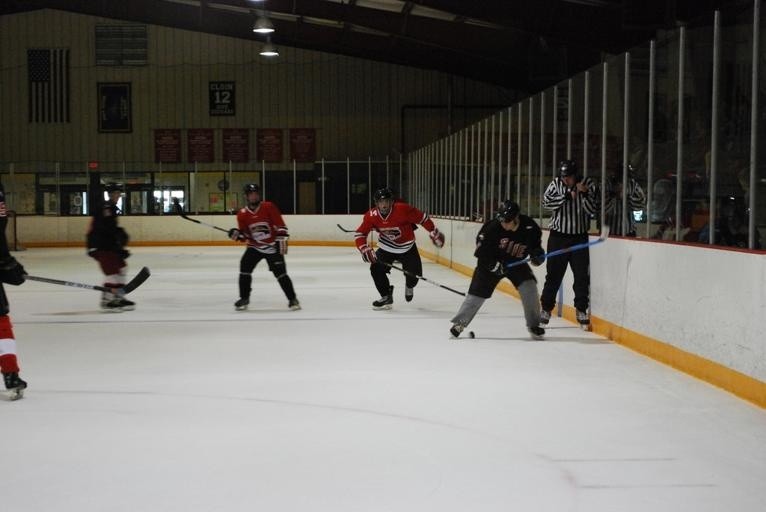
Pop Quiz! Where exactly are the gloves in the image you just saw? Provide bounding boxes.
[430,228,445,248]
[360,246,378,264]
[229,229,241,241]
[273,235,289,255]
[0,256,28,285]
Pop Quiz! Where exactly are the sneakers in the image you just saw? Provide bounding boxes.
[539,309,550,324]
[406,285,413,302]
[373,295,392,307]
[289,299,298,307]
[450,323,464,338]
[115,295,136,308]
[576,309,590,324]
[4,373,26,389]
[529,326,544,336]
[236,297,248,306]
[102,297,128,310]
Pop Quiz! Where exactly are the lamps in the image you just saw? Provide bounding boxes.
[252,14,276,35]
[257,34,280,58]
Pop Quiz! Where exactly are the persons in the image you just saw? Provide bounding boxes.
[481,97,766,249]
[228,183,300,307]
[539,160,600,324]
[87,183,135,311]
[170,197,183,214]
[449,199,545,338]
[0,182,28,391]
[354,187,445,307]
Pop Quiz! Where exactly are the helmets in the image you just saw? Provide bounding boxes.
[496,200,520,223]
[246,184,259,193]
[375,188,394,202]
[559,160,576,177]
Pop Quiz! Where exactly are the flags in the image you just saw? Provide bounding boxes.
[25,47,70,124]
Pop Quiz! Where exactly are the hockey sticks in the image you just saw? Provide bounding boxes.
[22,267,150,296]
[337,224,359,232]
[507,224,610,268]
[176,204,279,248]
